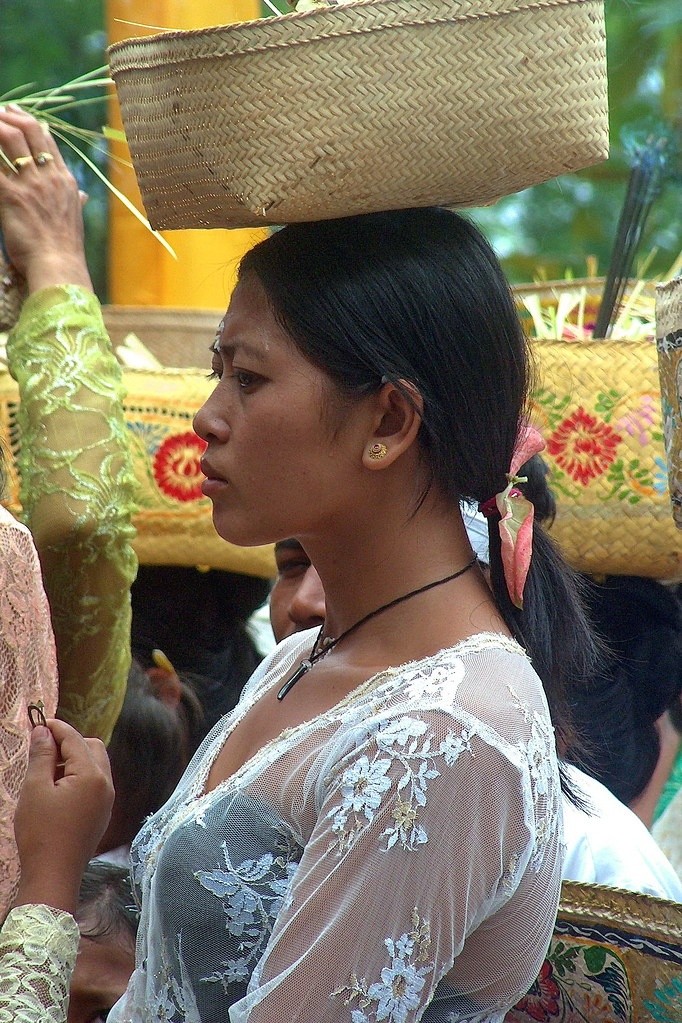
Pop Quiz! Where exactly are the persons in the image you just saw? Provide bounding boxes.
[105,206,565,1023]
[96,496,682,907]
[0,102,139,1023]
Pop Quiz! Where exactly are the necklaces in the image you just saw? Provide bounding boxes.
[277,552,479,700]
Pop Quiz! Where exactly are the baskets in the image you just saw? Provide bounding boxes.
[0,366,277,579]
[509,274,682,587]
[507,879,682,1023]
[105,0,610,233]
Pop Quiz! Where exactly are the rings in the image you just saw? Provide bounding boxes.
[34,153,54,164]
[11,156,32,170]
